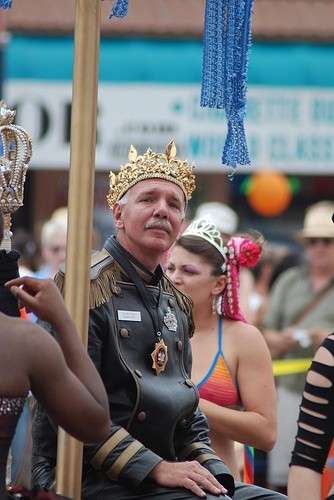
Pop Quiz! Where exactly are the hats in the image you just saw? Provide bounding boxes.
[196,201,239,237]
[295,201,334,236]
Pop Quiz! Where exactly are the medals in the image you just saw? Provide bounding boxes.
[151,338,168,376]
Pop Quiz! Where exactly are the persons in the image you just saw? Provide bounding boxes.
[0,275,111,500]
[11,205,97,485]
[287,332,334,500]
[29,162,287,500]
[194,201,334,490]
[165,218,277,483]
